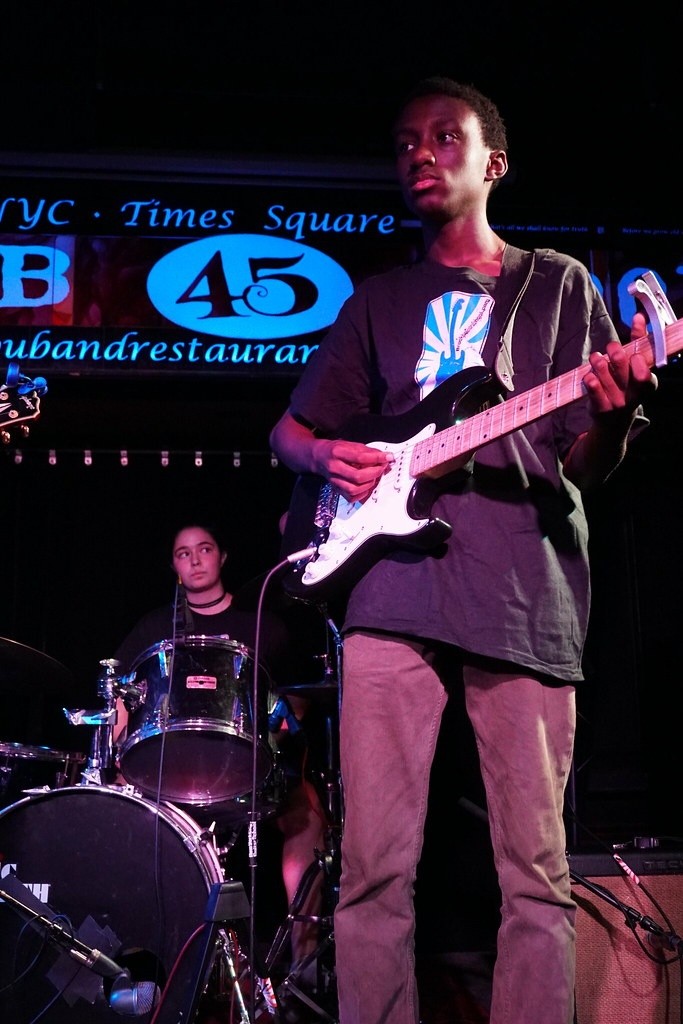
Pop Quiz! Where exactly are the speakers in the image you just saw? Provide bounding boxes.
[566,853,683,1024]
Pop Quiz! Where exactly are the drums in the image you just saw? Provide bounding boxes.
[0,628,289,1024]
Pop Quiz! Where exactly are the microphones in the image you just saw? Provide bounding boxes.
[647,931,683,950]
[110,968,162,1017]
[176,574,195,636]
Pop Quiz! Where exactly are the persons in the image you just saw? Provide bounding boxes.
[112,515,332,974]
[269,77,658,1024]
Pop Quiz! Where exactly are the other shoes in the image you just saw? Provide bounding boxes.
[274,978,318,1004]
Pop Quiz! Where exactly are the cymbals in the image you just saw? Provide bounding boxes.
[279,678,339,703]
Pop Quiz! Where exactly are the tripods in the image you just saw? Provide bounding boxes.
[251,601,342,1024]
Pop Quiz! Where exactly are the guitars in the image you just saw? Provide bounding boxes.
[296,316,683,585]
